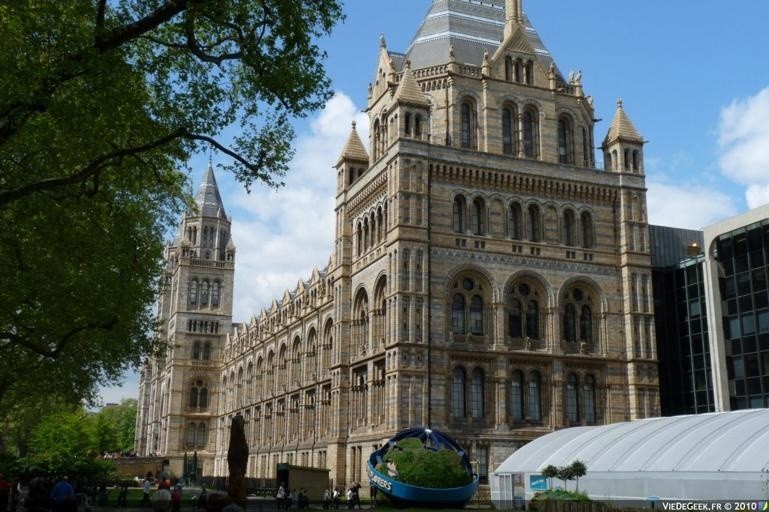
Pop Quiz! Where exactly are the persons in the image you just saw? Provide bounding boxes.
[386,456,399,481]
